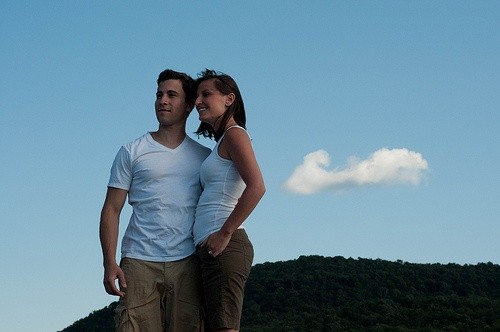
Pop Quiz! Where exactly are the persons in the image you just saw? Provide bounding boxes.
[193,68,266,332]
[99,69,212,332]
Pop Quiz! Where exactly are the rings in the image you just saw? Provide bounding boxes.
[208,250,213,254]
